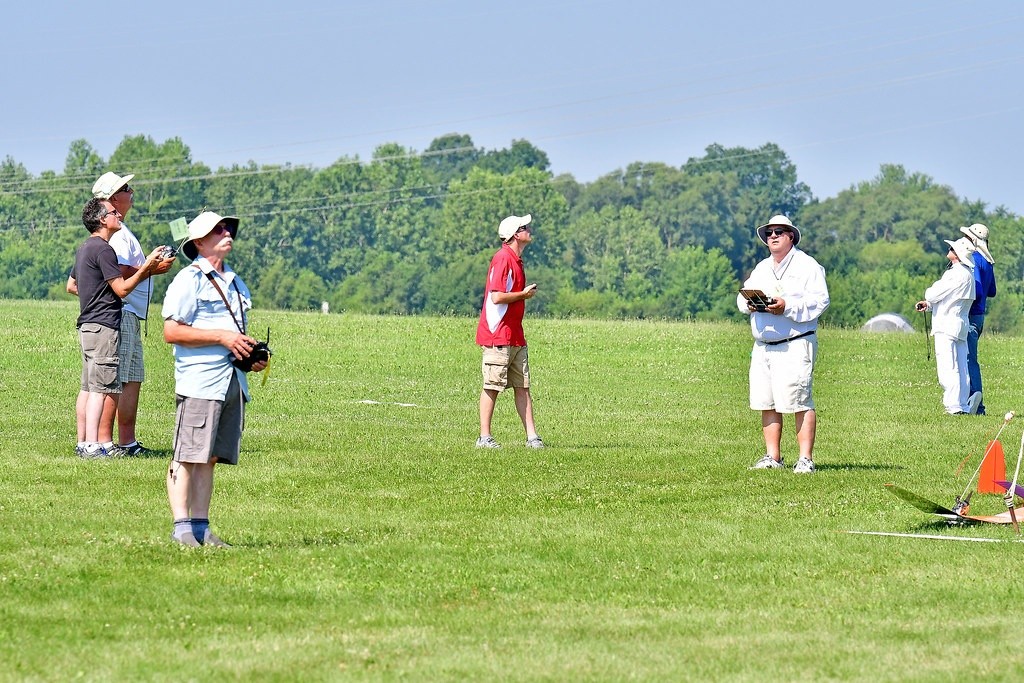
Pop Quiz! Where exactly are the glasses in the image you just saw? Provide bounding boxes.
[104,210,117,217]
[113,183,130,195]
[519,225,526,229]
[765,228,787,236]
[212,224,232,234]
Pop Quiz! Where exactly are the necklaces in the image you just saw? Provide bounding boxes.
[765,253,795,292]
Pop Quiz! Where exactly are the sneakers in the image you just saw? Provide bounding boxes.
[526,437,544,450]
[81,446,113,461]
[476,437,501,449]
[793,457,815,474]
[747,455,784,471]
[74,443,85,456]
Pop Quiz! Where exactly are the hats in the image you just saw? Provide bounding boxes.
[944,237,975,267]
[181,212,240,262]
[960,223,995,264]
[757,215,801,247]
[92,172,135,200]
[498,214,531,243]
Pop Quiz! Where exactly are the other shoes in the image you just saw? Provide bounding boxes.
[204,528,230,547]
[106,444,130,459]
[966,391,982,415]
[118,442,163,458]
[172,531,201,547]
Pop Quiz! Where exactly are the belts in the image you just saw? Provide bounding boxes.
[761,330,816,345]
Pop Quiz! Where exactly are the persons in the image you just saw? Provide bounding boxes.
[162,211,270,551]
[914,223,998,415]
[474,214,546,447]
[65,171,176,461]
[736,215,830,476]
